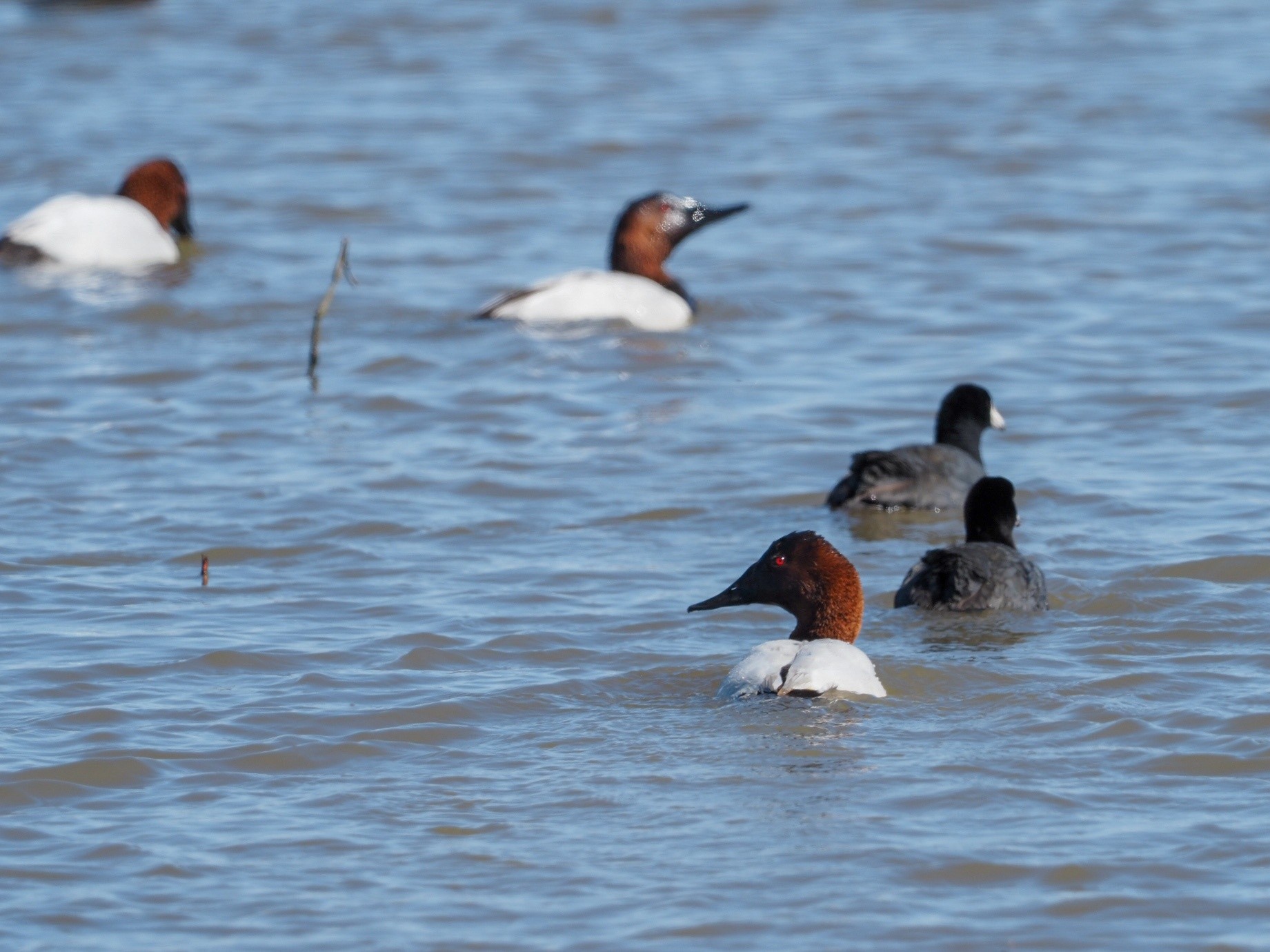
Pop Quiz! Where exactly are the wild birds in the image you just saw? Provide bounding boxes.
[474,189,750,332]
[894,477,1047,612]
[0,158,194,267]
[827,383,1006,509]
[689,529,888,698]
